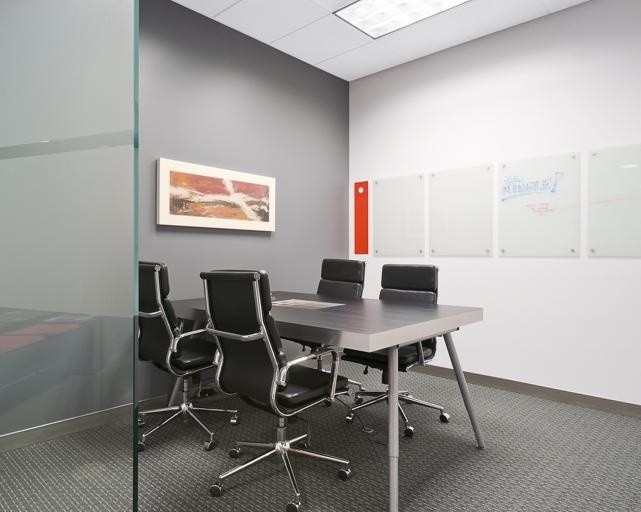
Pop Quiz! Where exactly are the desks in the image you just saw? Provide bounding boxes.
[169,290,484,512]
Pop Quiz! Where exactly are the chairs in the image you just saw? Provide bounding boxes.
[346,263,451,436]
[136,260,239,451]
[317,258,366,391]
[199,269,353,512]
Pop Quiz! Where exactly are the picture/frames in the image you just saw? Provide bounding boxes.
[156,157,276,233]
